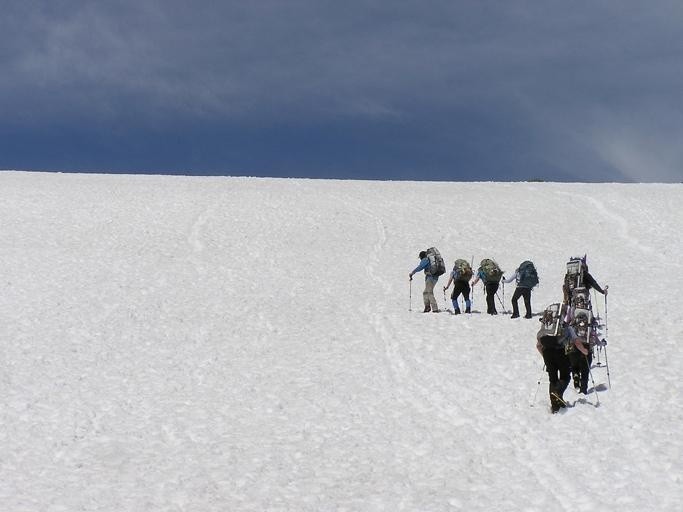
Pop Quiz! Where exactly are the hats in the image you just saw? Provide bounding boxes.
[417,250,427,260]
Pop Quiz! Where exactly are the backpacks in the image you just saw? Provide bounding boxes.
[538,301,568,351]
[479,258,503,283]
[452,258,474,278]
[568,307,595,347]
[425,246,446,277]
[569,286,592,311]
[517,260,540,288]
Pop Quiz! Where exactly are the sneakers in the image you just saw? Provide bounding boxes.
[550,405,560,414]
[573,375,580,389]
[549,389,567,408]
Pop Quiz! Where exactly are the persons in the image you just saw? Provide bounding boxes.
[442,258,474,315]
[501,260,538,319]
[565,295,607,394]
[470,258,502,315]
[409,247,445,313]
[560,277,599,320]
[534,302,589,414]
[561,255,607,302]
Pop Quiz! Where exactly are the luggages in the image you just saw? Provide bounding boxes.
[563,259,586,299]
